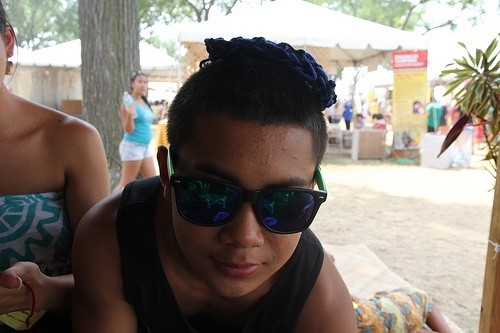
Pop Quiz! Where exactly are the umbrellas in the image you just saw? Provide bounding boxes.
[11,38,178,70]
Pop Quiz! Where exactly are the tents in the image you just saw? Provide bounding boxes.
[175,0,455,163]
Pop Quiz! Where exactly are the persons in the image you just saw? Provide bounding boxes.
[0,0,110,333]
[427,97,442,133]
[351,287,451,333]
[439,98,494,140]
[334,95,385,130]
[70,36,356,333]
[113,71,156,191]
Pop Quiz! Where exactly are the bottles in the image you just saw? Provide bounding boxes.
[123,91,138,119]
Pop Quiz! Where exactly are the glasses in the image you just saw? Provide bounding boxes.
[168,146,328,235]
